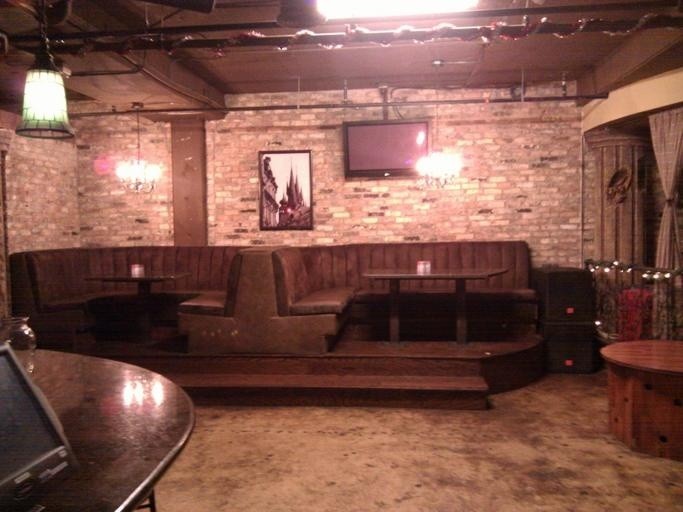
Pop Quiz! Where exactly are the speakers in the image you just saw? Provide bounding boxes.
[536,321,596,374]
[530,266,595,322]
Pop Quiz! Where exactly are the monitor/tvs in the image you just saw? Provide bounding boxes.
[343,119,432,180]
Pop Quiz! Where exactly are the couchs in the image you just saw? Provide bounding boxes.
[267,242,540,355]
[20,246,256,336]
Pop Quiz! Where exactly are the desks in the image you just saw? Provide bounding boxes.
[88,268,190,337]
[598,338,682,463]
[359,260,509,343]
[18,347,196,509]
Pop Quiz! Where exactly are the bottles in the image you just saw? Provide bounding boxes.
[0,315,36,376]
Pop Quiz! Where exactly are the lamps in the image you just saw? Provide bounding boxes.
[113,102,162,194]
[15,0,77,140]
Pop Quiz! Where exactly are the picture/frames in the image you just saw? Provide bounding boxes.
[258,151,313,231]
